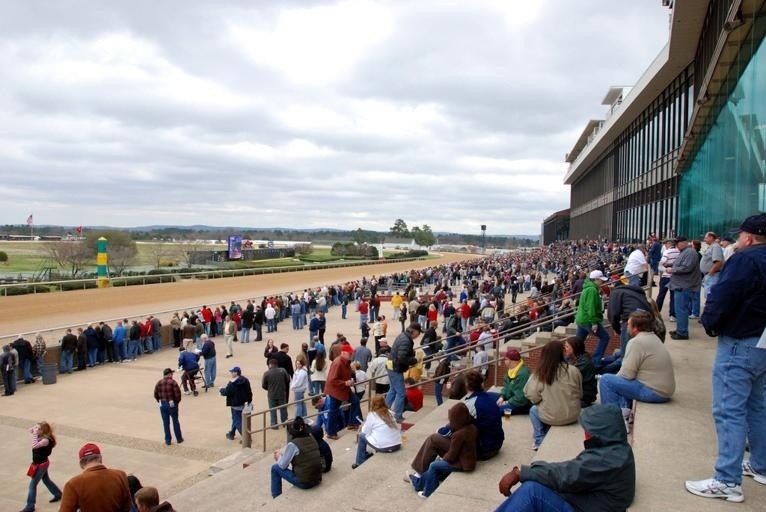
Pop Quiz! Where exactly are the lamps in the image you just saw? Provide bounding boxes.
[697,91,711,106]
[723,7,745,31]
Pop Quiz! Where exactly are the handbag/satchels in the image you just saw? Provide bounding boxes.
[27,463,37,477]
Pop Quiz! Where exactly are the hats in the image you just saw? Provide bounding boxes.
[410,322,426,333]
[79,442,100,460]
[378,337,387,342]
[673,236,686,243]
[342,344,354,353]
[500,350,521,361]
[230,366,242,373]
[164,368,175,375]
[729,214,766,236]
[287,416,313,437]
[590,270,608,281]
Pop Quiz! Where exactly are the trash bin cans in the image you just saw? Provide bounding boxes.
[39,363,58,384]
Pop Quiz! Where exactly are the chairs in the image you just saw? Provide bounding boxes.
[179,364,207,396]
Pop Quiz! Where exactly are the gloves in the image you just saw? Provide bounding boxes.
[498,466,521,497]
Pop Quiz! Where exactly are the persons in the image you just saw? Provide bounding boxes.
[213,263,450,357]
[171,305,216,394]
[217,323,426,498]
[20,423,62,512]
[2,314,162,397]
[58,443,175,512]
[155,368,185,444]
[436,234,701,364]
[404,310,674,512]
[684,214,766,503]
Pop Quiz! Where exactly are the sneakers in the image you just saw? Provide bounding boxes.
[226,433,233,440]
[684,478,744,503]
[403,471,428,499]
[669,316,676,321]
[688,314,699,319]
[48,495,61,502]
[669,330,689,340]
[742,459,766,484]
[20,508,35,512]
